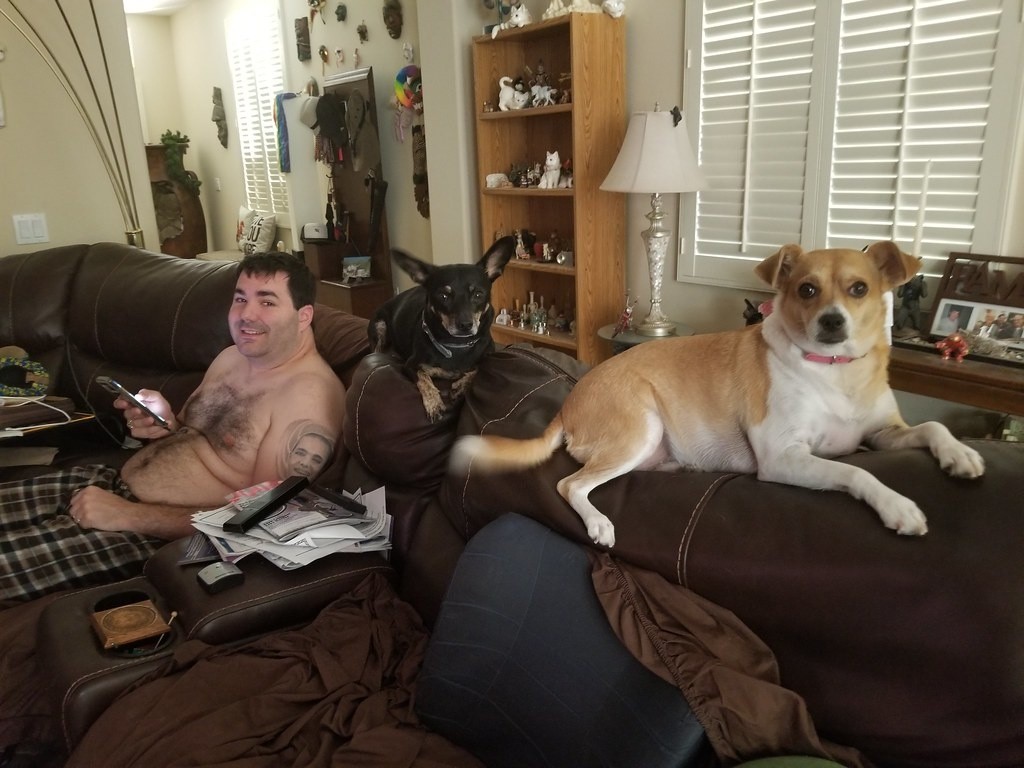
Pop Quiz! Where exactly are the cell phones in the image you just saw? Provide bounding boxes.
[96,376,167,427]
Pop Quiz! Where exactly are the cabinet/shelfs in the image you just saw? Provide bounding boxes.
[474,14,625,370]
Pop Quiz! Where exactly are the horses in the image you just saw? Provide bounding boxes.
[531,85,558,108]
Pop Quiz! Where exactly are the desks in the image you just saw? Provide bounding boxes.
[887,345,1024,419]
[599,322,696,355]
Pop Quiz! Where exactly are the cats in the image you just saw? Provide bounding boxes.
[498,76,528,112]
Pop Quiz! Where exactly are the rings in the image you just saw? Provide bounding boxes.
[125,419,135,430]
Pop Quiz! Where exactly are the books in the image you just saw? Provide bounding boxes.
[176,476,392,567]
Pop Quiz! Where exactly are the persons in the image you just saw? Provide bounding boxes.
[742,295,768,327]
[969,312,1024,346]
[891,274,928,331]
[937,308,961,335]
[1,251,346,600]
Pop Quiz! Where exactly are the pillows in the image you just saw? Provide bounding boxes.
[243,212,277,256]
[236,205,257,254]
[414,513,718,767]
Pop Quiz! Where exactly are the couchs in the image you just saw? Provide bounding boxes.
[0,242,1024,768]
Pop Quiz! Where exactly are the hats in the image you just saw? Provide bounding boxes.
[300,223,330,243]
[299,97,320,137]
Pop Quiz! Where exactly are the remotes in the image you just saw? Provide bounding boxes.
[310,482,366,514]
[223,475,309,534]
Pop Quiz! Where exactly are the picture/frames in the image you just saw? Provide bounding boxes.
[921,251,1024,354]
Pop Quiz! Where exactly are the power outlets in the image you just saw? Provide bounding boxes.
[214,178,219,191]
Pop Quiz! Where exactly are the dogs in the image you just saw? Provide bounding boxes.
[446,242,984,547]
[492,4,532,40]
[366,235,518,426]
[600,0,625,19]
[538,150,561,189]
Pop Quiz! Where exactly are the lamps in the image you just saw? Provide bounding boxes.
[599,110,708,335]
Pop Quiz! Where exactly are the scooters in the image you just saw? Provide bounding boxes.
[612,290,639,339]
